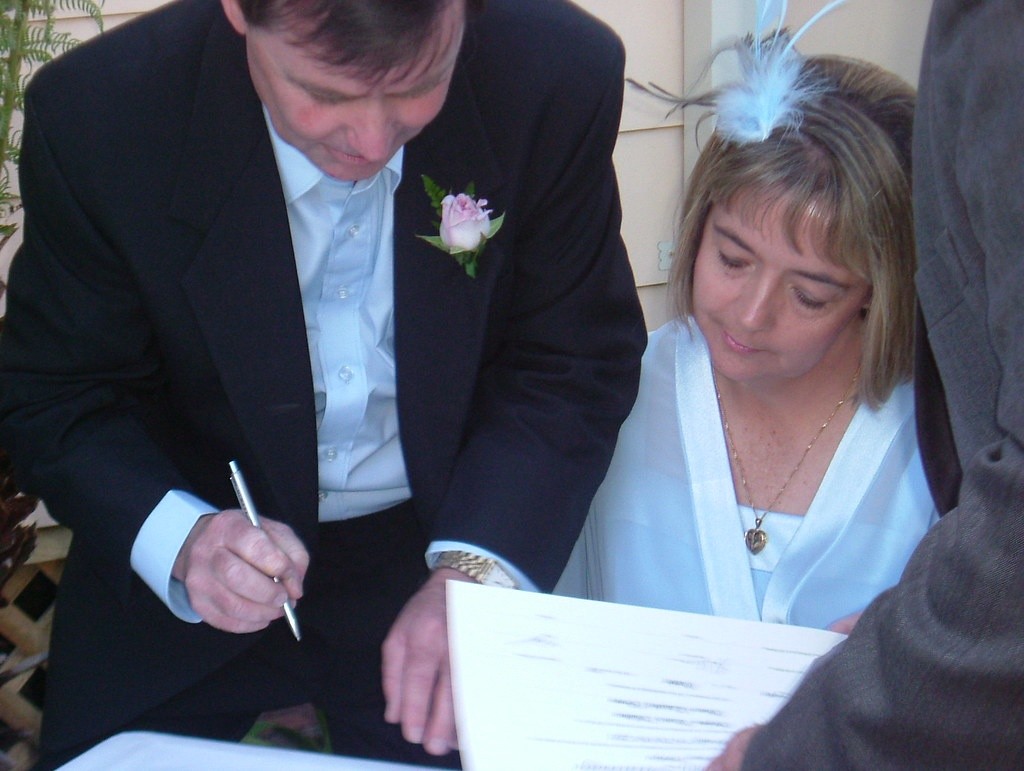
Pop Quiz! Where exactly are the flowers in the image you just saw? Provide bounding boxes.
[406,175,506,279]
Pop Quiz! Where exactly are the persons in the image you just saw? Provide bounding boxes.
[703,1,1024,771]
[0,0,649,771]
[552,53,940,637]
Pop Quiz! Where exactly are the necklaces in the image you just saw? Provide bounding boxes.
[712,367,861,555]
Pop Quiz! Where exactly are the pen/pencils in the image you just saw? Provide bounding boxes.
[227,461,301,642]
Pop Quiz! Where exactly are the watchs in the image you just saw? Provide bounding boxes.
[431,550,521,590]
